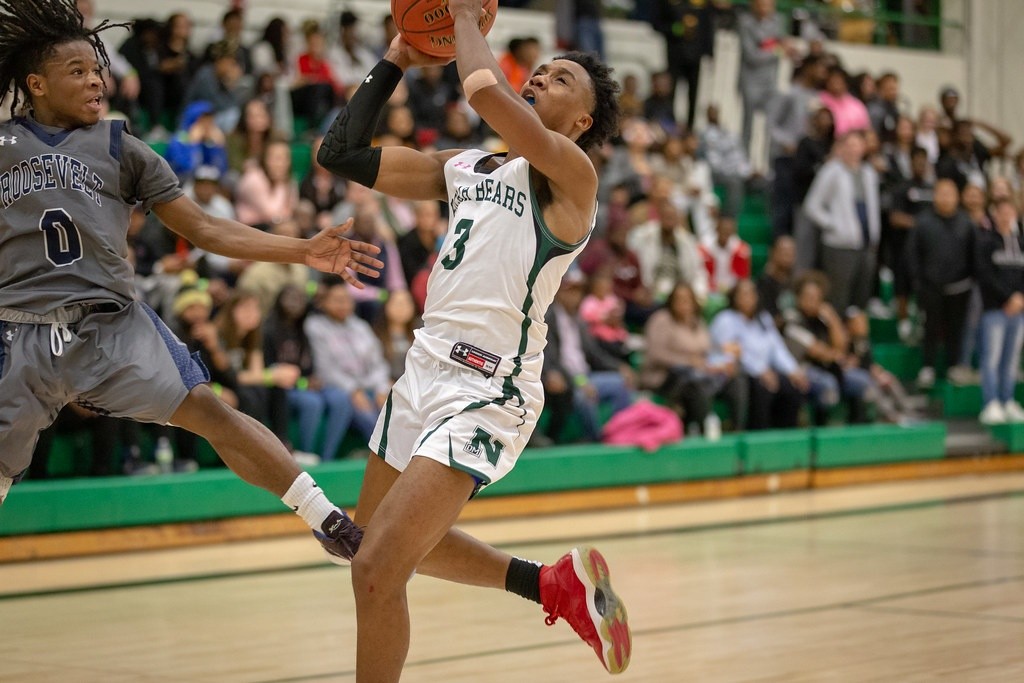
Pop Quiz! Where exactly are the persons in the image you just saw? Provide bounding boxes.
[317,0,632,683]
[0,1,385,566]
[32,0,1024,484]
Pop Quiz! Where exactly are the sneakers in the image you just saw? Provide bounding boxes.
[310,509,416,583]
[540,546,631,675]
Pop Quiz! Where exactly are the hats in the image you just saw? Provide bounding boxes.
[170,269,212,315]
[562,271,584,289]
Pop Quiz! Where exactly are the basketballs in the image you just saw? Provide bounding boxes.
[391,0,499,58]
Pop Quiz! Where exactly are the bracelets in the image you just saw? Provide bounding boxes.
[211,382,223,395]
[196,277,209,290]
[572,374,589,385]
[174,129,190,145]
[121,68,138,76]
[773,46,781,57]
[263,368,272,387]
[296,376,309,391]
[378,288,389,302]
[672,21,684,36]
[306,279,317,301]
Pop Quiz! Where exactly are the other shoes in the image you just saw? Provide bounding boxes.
[1002,397,1023,422]
[978,399,1007,426]
